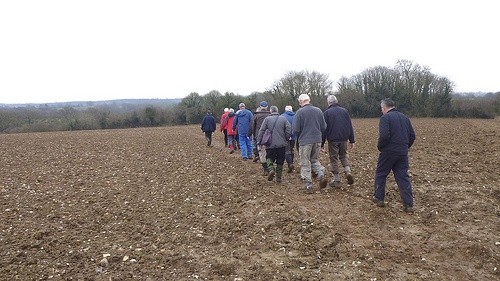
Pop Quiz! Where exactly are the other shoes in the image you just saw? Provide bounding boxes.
[207,141,211,146]
[403,202,413,212]
[287,163,294,173]
[305,183,314,194]
[237,144,241,149]
[261,163,269,176]
[253,154,260,162]
[346,171,354,184]
[267,170,275,181]
[319,177,327,189]
[276,175,282,183]
[372,199,385,206]
[230,149,234,154]
[329,175,341,187]
[234,146,237,150]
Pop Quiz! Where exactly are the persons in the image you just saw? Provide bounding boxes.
[291,93,327,194]
[201,111,216,146]
[321,95,356,187]
[372,98,415,214]
[219,101,296,183]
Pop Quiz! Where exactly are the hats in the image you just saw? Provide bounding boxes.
[259,101,268,107]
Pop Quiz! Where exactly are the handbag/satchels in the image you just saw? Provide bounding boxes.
[258,114,279,145]
